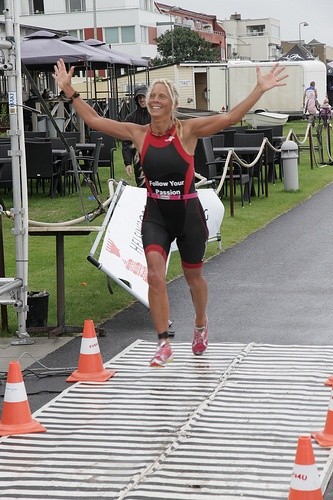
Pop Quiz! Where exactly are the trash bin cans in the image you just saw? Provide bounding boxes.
[26,291,49,332]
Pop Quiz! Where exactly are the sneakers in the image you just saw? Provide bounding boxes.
[150,340,173,367]
[192,317,208,354]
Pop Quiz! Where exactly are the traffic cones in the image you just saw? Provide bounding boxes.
[0,360,47,436]
[65,319,116,382]
[286,435,326,500]
[311,384,333,449]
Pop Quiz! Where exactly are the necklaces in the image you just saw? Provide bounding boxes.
[149,121,173,137]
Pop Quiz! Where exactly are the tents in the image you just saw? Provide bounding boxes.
[273,44,333,76]
[19,31,152,98]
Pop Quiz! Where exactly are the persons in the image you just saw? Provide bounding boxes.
[41,89,55,113]
[54,90,71,132]
[303,81,319,127]
[52,58,288,367]
[317,97,332,127]
[122,85,152,188]
[24,89,40,131]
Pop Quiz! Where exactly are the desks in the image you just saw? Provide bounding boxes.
[213,147,264,199]
[76,144,104,187]
[53,150,72,196]
[274,136,287,180]
[11,227,108,336]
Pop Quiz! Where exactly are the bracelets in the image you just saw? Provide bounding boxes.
[67,91,80,104]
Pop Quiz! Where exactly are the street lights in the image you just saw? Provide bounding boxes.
[299,21,309,42]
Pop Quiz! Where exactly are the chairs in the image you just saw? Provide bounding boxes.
[194,125,284,217]
[0,131,117,199]
